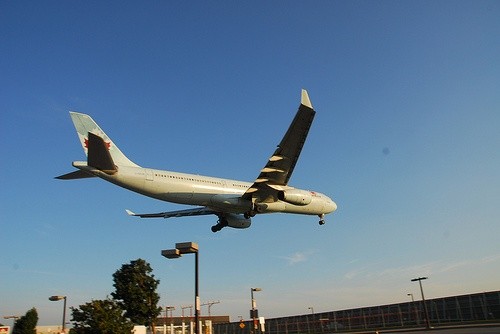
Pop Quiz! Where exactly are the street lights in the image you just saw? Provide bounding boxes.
[48,295,67,333]
[161,241,200,334]
[411,277,434,329]
[407,293,414,302]
[250,287,262,333]
[309,306,314,314]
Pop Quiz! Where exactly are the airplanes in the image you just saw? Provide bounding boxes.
[54,89,338,232]
